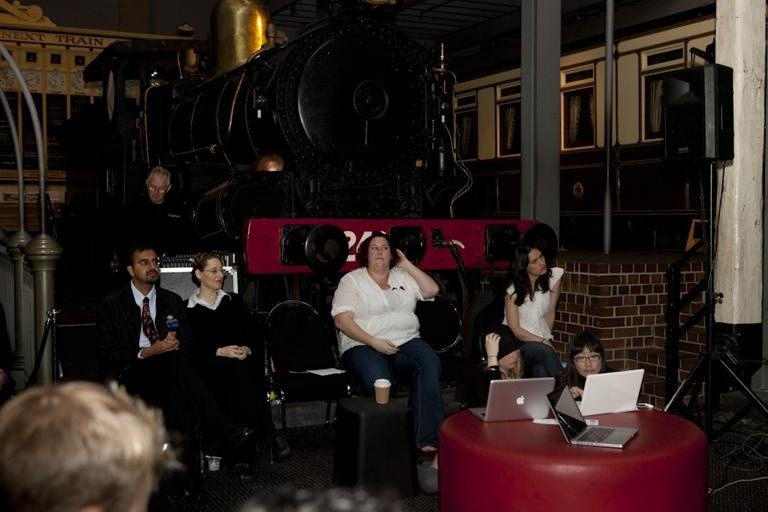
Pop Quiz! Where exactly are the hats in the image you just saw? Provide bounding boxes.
[479,323,525,360]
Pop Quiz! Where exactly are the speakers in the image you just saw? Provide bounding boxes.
[659,63,734,162]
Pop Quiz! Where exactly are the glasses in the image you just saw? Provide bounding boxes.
[147,181,168,193]
[572,354,600,363]
[131,259,161,266]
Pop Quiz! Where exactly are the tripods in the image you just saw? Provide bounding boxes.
[665,164,768,442]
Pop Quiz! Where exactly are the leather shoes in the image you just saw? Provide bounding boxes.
[263,430,289,459]
[211,423,254,451]
[230,460,257,482]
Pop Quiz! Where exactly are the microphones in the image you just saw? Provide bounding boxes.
[165,313,178,331]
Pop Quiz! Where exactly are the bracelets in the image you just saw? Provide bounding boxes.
[487,355,497,359]
[540,337,546,344]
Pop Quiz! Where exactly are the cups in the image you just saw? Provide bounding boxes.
[208,457,221,470]
[373,378,391,404]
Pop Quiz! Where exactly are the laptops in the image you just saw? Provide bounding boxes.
[575,368,646,416]
[546,383,640,449]
[467,376,558,423]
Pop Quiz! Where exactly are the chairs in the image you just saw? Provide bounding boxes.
[261,299,349,435]
[50,305,104,391]
[414,290,470,410]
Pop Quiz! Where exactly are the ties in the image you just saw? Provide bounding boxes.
[141,297,157,346]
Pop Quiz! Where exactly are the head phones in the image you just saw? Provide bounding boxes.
[357,232,399,270]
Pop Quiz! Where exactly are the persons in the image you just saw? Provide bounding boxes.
[466,324,534,409]
[554,330,618,401]
[330,233,443,454]
[0,379,185,512]
[493,241,566,388]
[179,251,291,484]
[123,166,194,255]
[102,243,256,504]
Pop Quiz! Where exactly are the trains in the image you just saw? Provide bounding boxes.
[65,3,538,378]
[433,19,715,242]
[2,6,210,247]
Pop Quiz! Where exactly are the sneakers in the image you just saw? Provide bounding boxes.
[416,455,438,493]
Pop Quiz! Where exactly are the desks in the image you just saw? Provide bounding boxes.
[331,395,421,500]
[440,397,711,512]
